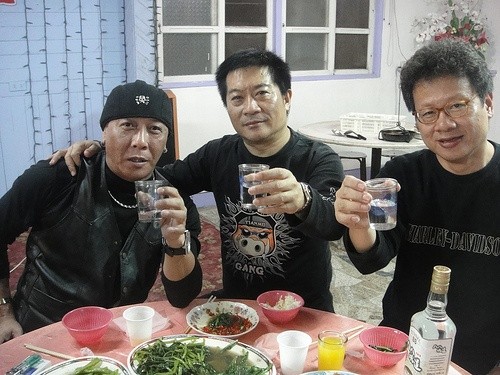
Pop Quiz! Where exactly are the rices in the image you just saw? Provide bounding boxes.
[259,295,300,310]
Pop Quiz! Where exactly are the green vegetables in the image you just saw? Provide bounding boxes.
[132,336,220,375]
[220,339,273,375]
[70,357,120,374]
[209,307,243,327]
[368,341,408,352]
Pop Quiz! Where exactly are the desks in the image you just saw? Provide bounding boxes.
[297,121,426,179]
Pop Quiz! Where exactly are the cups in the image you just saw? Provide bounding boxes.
[239,164,270,209]
[277,329,312,375]
[317,330,349,370]
[123,306,155,346]
[364,178,398,232]
[134,179,168,224]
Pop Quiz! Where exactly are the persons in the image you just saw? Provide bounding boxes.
[47,47,346,313]
[0,79,203,346]
[333,39,500,375]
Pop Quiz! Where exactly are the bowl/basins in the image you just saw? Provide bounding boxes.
[358,326,408,366]
[62,306,114,345]
[186,301,260,339]
[257,290,304,324]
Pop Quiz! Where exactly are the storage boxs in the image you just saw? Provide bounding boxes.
[340,112,406,138]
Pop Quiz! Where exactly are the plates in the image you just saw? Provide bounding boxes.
[37,357,130,375]
[300,371,359,375]
[127,334,276,375]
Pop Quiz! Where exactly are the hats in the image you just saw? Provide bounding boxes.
[99,79,172,131]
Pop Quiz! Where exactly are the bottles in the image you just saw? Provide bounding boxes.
[405,266,457,375]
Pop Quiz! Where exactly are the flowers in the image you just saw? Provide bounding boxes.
[409,0,496,54]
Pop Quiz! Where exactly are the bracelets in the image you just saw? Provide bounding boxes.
[0,297,14,305]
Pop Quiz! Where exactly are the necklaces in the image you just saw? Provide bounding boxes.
[108,171,156,209]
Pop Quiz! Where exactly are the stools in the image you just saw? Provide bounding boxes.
[337,151,367,181]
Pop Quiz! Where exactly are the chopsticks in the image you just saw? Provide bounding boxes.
[309,325,367,359]
[184,295,216,334]
[24,344,76,360]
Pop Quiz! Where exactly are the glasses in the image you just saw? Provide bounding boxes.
[412,94,478,124]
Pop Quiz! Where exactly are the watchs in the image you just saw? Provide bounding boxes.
[161,231,191,257]
[295,182,313,212]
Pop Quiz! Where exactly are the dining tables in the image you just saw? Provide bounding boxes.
[0,298,472,375]
